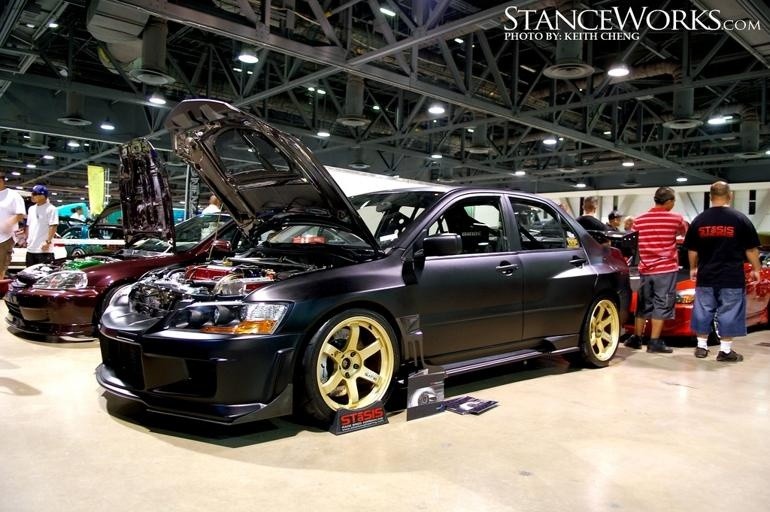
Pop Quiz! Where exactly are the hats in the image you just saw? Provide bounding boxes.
[608,212,623,220]
[29,185,49,197]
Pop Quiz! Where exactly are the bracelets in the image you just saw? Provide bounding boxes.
[46,240,52,244]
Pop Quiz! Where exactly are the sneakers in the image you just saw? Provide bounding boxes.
[624,335,642,349]
[647,340,673,354]
[716,350,743,362]
[695,347,709,358]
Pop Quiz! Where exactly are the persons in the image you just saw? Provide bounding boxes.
[0,173,27,279]
[622,216,633,231]
[574,197,611,245]
[69,206,86,225]
[202,195,220,213]
[626,187,689,352]
[605,210,624,231]
[684,181,761,360]
[24,185,59,267]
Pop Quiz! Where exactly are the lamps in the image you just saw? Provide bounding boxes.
[427,98,445,115]
[149,91,166,106]
[67,139,82,149]
[621,159,635,167]
[607,59,630,78]
[239,47,259,65]
[676,175,688,184]
[707,111,726,126]
[429,151,442,159]
[100,119,115,132]
[5,155,112,206]
[543,134,556,147]
[514,170,525,175]
[576,181,586,189]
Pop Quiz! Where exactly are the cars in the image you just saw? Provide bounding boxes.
[4,136,243,342]
[624,232,769,339]
[96,89,630,439]
